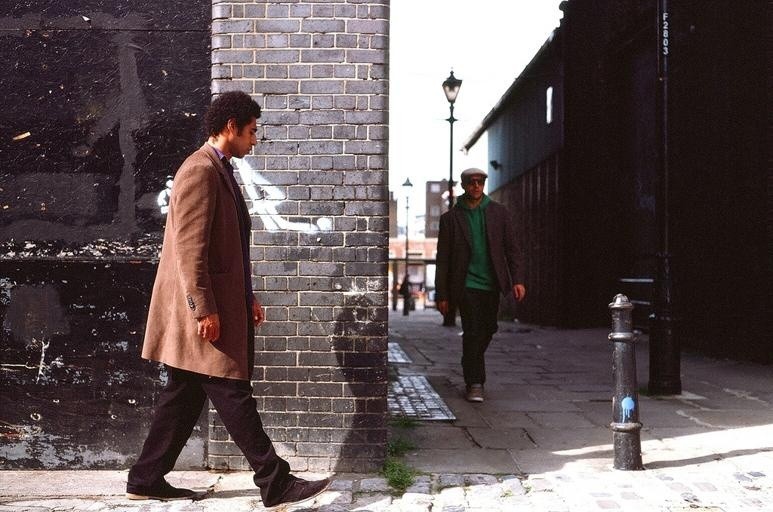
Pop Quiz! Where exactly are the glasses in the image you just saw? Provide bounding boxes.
[466,178,485,185]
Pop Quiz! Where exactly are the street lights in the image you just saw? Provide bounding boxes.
[442,66,463,207]
[401,177,412,274]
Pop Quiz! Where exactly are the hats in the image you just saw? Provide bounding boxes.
[461,168,487,180]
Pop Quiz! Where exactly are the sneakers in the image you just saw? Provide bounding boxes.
[127,484,195,500]
[266,478,331,512]
[465,383,484,402]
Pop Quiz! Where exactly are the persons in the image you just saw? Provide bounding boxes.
[434,167,526,402]
[402,274,409,316]
[126,90,332,511]
